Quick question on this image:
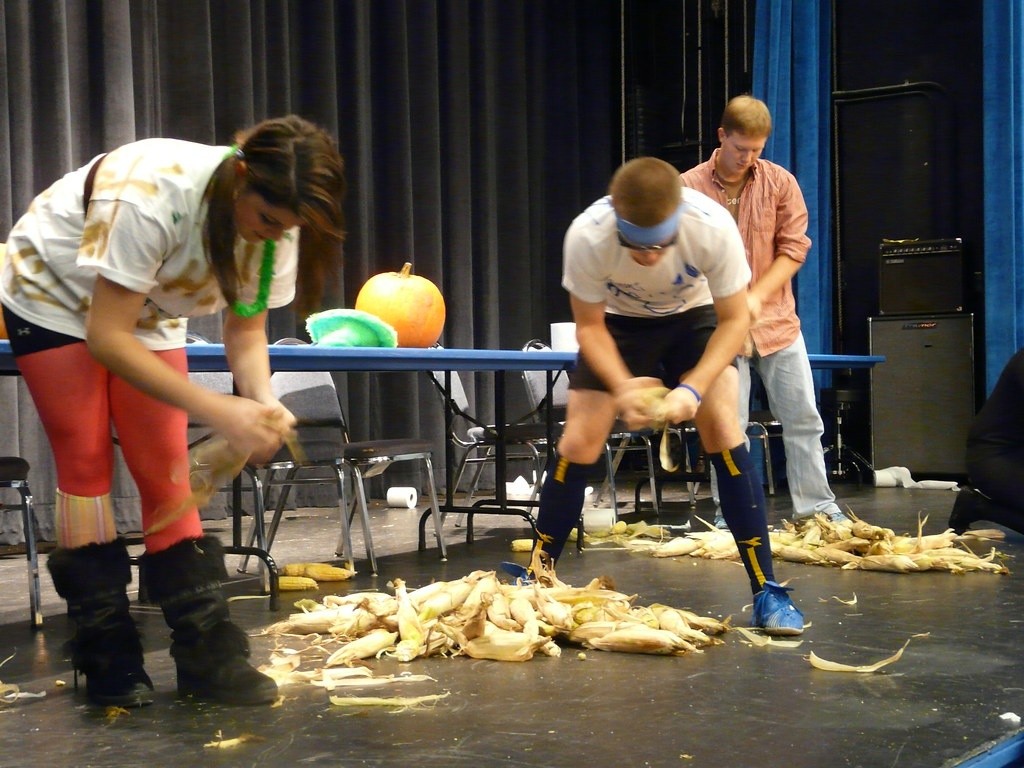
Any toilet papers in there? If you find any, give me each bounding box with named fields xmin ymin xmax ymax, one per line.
xmin=873 ymin=469 xmax=898 ymax=488
xmin=386 ymin=486 xmax=419 ymax=508
xmin=550 ymin=322 xmax=580 ymax=352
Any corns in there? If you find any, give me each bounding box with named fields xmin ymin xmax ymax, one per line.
xmin=510 ymin=516 xmax=1013 ymax=572
xmin=269 ymin=561 xmax=724 ymax=664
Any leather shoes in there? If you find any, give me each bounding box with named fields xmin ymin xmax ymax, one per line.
xmin=948 ymin=487 xmax=977 ymax=535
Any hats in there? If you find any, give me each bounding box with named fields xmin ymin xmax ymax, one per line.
xmin=305 ymin=308 xmax=398 ymax=348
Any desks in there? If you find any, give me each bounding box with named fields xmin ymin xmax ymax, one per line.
xmin=0 ymin=340 xmax=895 ymax=612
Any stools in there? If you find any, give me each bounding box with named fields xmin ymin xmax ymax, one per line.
xmin=821 ymin=385 xmax=871 ymax=491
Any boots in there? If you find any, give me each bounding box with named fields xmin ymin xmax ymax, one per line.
xmin=137 ymin=535 xmax=279 ymax=706
xmin=47 ymin=535 xmax=152 ymax=705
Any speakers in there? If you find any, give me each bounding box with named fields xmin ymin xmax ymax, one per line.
xmin=879 ymin=237 xmax=966 ymax=316
xmin=869 ymin=314 xmax=976 ymax=476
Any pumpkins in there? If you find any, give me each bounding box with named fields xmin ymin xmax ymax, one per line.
xmin=356 ymin=262 xmax=446 ymax=348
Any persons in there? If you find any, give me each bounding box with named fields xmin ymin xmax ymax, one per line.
xmin=0 ymin=115 xmax=348 ymax=709
xmin=948 ymin=347 xmax=1024 ymax=535
xmin=500 ymin=158 xmax=805 ymax=636
xmin=678 ymin=96 xmax=850 ymax=525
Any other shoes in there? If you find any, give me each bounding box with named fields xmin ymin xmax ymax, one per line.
xmin=828 ymin=511 xmax=848 ymax=523
xmin=498 ymin=561 xmax=536 ymax=585
xmin=714 ymin=516 xmax=728 ymax=528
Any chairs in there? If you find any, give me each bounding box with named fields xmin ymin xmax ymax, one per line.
xmin=0 ymin=312 xmax=872 ymax=630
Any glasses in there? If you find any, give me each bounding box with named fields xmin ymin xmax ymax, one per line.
xmin=617 ymin=231 xmax=674 ymax=251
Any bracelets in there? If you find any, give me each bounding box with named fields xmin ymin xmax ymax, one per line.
xmin=677 ymin=384 xmax=701 ymax=406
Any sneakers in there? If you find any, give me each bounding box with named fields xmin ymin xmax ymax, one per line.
xmin=752 ymin=581 xmax=803 ymax=634
